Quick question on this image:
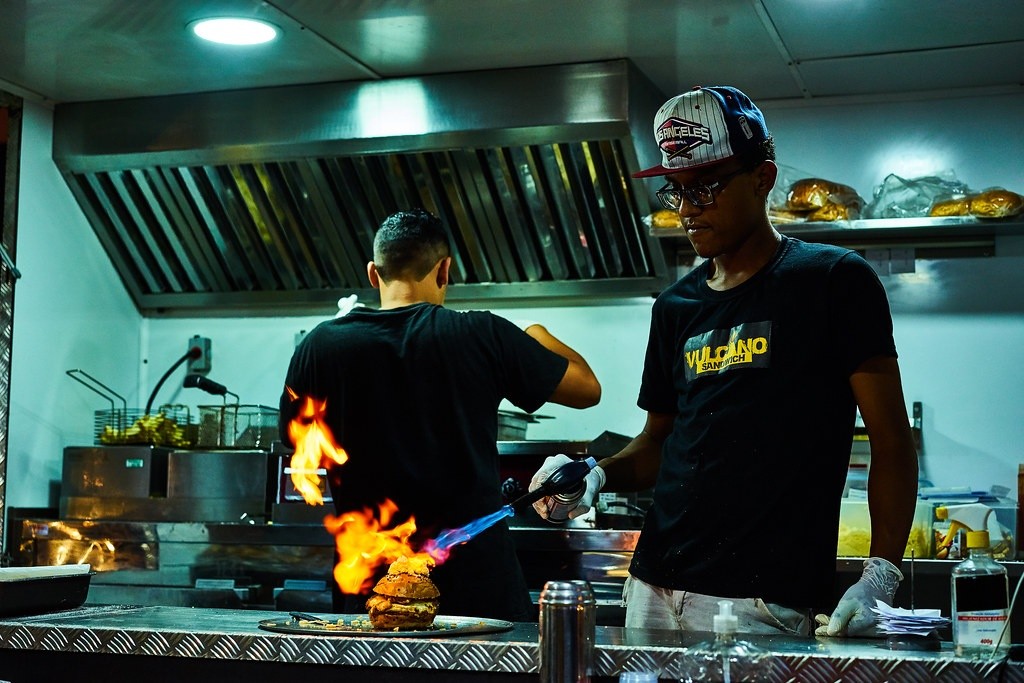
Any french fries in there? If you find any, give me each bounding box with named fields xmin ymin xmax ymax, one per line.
xmin=100 ymin=415 xmax=193 ymax=446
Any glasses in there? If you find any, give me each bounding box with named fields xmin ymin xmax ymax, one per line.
xmin=656 ymin=161 xmax=761 ymax=210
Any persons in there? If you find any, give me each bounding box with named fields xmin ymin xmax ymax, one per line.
xmin=279 ymin=209 xmax=600 ymax=622
xmin=527 ymin=87 xmax=920 ymax=638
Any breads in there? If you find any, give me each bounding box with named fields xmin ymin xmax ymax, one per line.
xmin=771 ymin=178 xmax=862 ymax=220
xmin=930 ymin=190 xmax=1024 ymax=217
xmin=653 ymin=210 xmax=681 ymax=228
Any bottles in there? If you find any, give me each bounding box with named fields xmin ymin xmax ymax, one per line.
xmin=680 ymin=600 xmax=773 ymax=683
xmin=620 ymin=672 xmax=658 ymax=683
xmin=848 ymin=464 xmax=868 ymax=491
xmin=952 ymin=532 xmax=1011 ymax=659
xmin=538 ymin=581 xmax=596 ymax=683
xmin=932 ymin=508 xmax=967 ymax=560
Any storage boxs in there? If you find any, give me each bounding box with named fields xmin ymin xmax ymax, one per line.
xmin=0 ymin=571 xmax=97 ymax=619
xmin=945 ymin=504 xmax=1021 ymax=559
xmin=834 ymin=501 xmax=936 ymax=559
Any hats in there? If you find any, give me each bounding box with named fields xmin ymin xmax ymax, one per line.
xmin=630 ymin=85 xmax=770 ymax=178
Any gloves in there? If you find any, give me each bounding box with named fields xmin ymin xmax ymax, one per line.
xmin=528 ymin=453 xmax=607 ymax=521
xmin=813 ymin=558 xmax=904 ymax=637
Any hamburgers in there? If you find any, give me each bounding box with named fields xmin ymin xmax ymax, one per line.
xmin=364 ymin=574 xmax=440 ymax=629
